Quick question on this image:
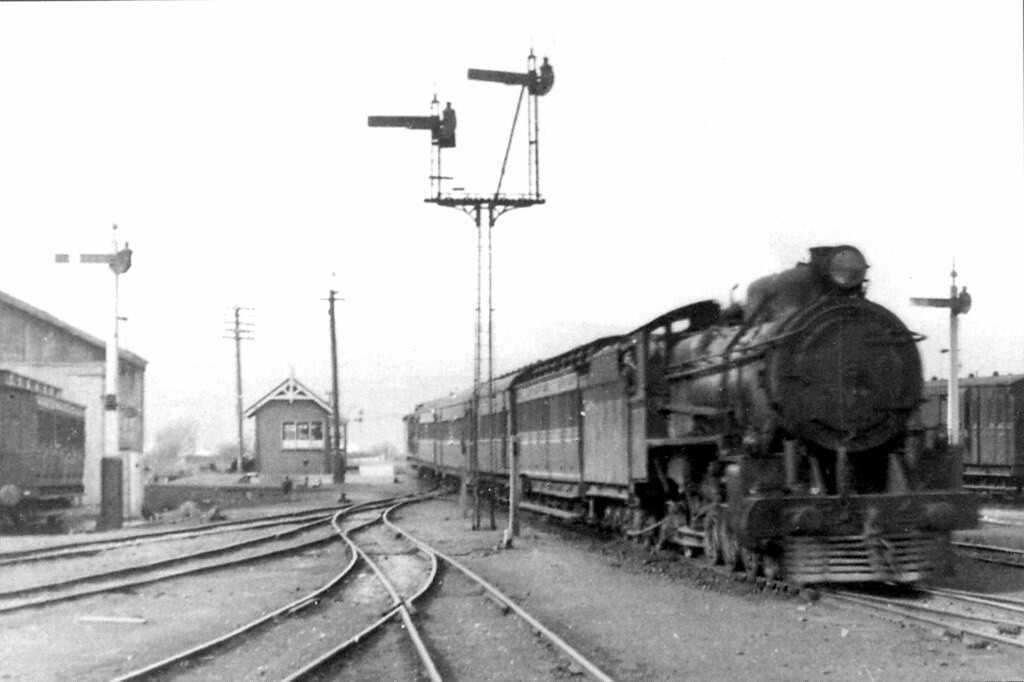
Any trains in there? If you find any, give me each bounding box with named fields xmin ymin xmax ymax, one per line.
xmin=922 ymin=372 xmax=1024 ymax=510
xmin=405 ymin=245 xmax=983 ymax=593
